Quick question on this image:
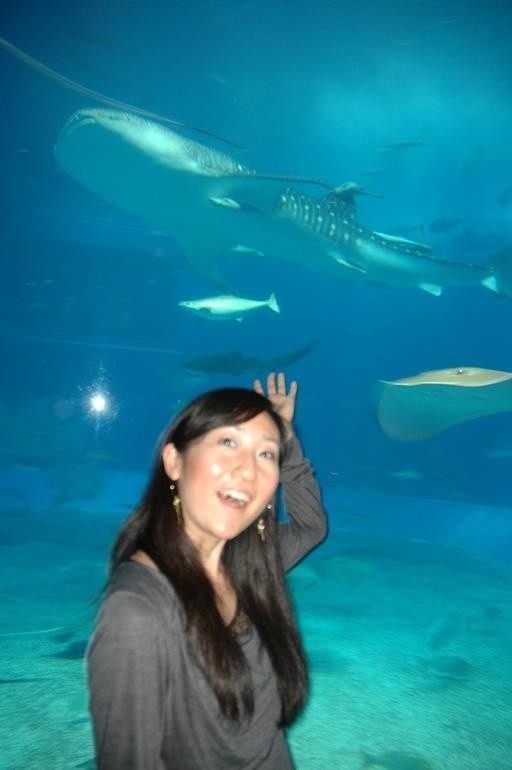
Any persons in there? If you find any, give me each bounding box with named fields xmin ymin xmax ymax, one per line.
xmin=84 ymin=373 xmax=331 ymax=770
